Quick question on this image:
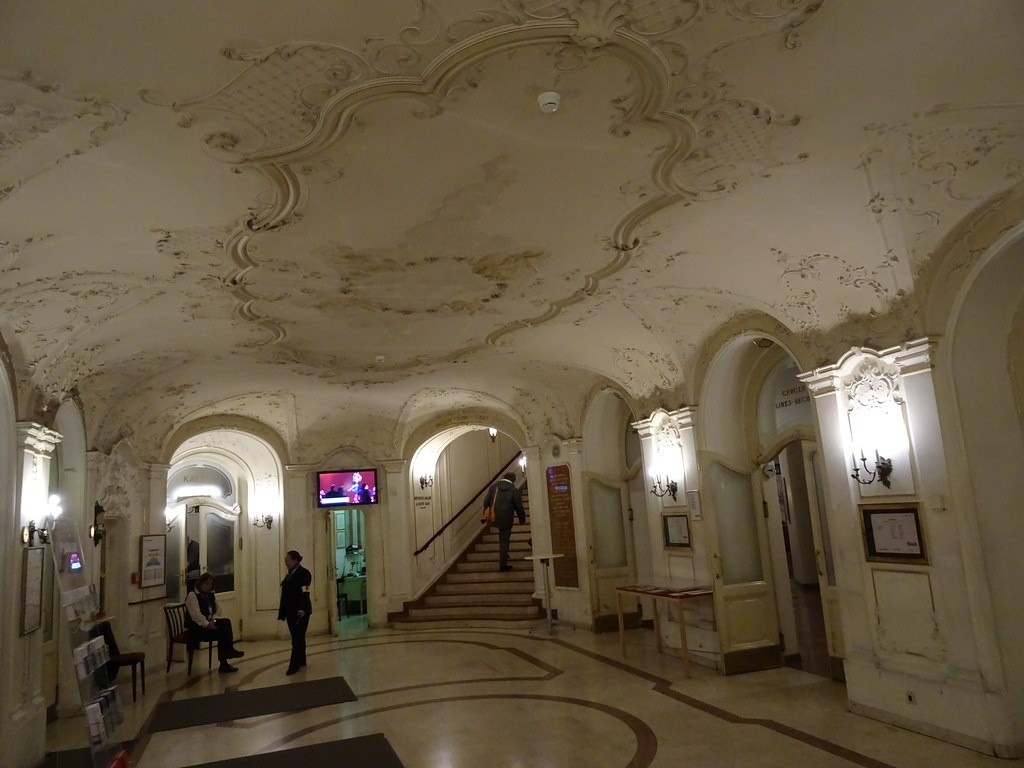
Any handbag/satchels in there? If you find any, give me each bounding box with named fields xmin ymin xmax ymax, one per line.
xmin=480 ymin=507 xmax=496 ymax=527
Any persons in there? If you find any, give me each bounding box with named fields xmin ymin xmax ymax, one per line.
xmin=184 ymin=572 xmax=245 ymax=672
xmin=278 ymin=550 xmax=312 ymax=675
xmin=325 ymin=484 xmax=337 ymax=496
xmin=334 ymin=487 xmax=346 ymax=497
xmin=187 ymin=533 xmax=199 ymax=571
xmin=357 ymin=483 xmax=371 ymax=502
xmin=483 ymin=471 xmax=526 ymax=571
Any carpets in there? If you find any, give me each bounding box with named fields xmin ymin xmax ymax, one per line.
xmin=182 ymin=732 xmax=403 ymax=768
xmin=147 ymin=676 xmax=358 ymax=734
xmin=34 ymin=739 xmax=135 ymax=768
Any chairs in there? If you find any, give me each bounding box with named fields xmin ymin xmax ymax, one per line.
xmin=163 ymin=604 xmax=212 ymax=675
xmin=87 ymin=621 xmax=146 ymax=703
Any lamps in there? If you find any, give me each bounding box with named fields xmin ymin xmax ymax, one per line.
xmin=22 ymin=495 xmax=63 ymax=545
xmin=650 ymin=473 xmax=677 ymax=502
xmin=518 ymin=456 xmax=527 ymax=480
xmin=851 ymin=448 xmax=892 ymax=489
xmin=488 ymin=427 xmax=497 ymax=444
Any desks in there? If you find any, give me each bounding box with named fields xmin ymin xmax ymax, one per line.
xmin=524 ymin=554 xmax=576 ymax=636
xmin=615 ymin=585 xmax=714 ymax=679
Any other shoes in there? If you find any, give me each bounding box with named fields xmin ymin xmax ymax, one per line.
xmin=219 ymin=664 xmax=239 ymax=673
xmin=227 ymin=648 xmax=245 ymax=659
xmin=286 ymin=665 xmax=299 ymax=675
xmin=499 ymin=565 xmax=513 ymax=572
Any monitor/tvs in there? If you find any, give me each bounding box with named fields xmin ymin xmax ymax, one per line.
xmin=316 ymin=468 xmax=378 ymax=508
